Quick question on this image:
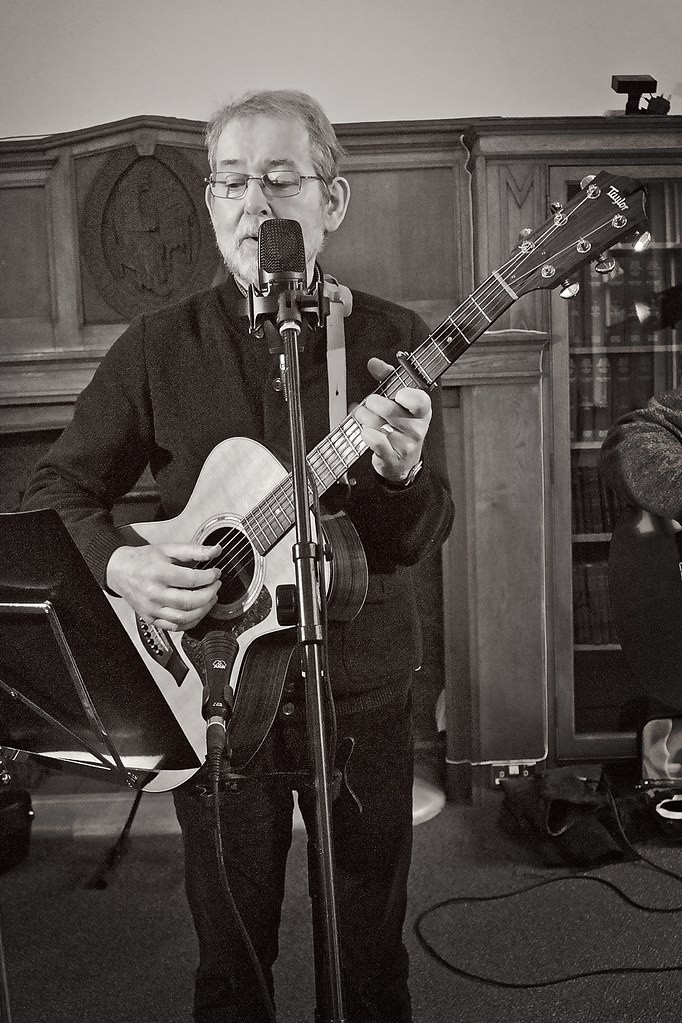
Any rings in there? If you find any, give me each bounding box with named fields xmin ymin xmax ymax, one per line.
xmin=379 ymin=424 xmax=393 ymax=437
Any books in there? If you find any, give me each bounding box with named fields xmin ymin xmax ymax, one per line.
xmin=568 ymin=258 xmax=664 ymax=648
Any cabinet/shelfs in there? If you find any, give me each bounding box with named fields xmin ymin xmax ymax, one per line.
xmin=0 ymin=112 xmax=682 ymax=812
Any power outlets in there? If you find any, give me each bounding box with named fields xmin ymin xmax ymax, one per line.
xmin=489 ymin=762 xmax=537 ymax=787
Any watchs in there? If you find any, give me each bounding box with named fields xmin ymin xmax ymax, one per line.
xmin=398 ymin=460 xmax=423 ymax=487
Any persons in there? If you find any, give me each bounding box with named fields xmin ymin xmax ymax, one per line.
xmin=598 ymin=389 xmax=682 ymax=520
xmin=19 ymin=88 xmax=455 ymax=1023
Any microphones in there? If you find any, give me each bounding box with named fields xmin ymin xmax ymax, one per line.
xmin=200 ymin=630 xmax=239 ymax=781
xmin=258 ymin=219 xmax=307 ymax=400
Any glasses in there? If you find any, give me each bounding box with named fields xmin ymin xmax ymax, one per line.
xmin=204 ymin=170 xmax=329 ymax=200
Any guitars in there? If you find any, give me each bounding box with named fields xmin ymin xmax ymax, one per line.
xmin=34 ymin=166 xmax=654 ymax=795
xmin=605 ymin=504 xmax=682 ymax=712
